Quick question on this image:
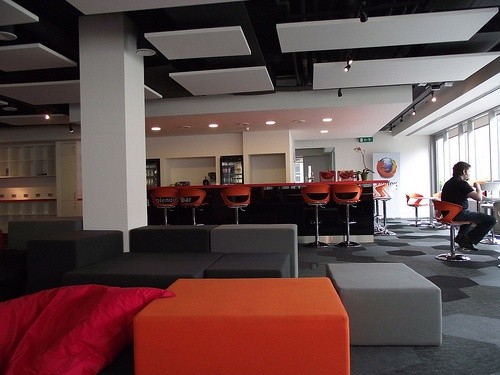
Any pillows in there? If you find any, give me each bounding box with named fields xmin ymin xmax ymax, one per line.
xmin=0 ymin=284 xmax=177 ymax=375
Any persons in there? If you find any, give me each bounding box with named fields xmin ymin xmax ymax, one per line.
xmin=441 ymin=161 xmax=496 ymax=251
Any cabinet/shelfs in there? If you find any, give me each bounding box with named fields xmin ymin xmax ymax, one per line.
xmin=0 ymin=143 xmax=57 ymax=233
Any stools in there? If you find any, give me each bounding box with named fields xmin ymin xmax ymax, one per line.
xmin=325 ymin=263 xmax=442 ymax=346
xmin=151 ymin=184 xmax=500 ymax=262
xmin=133 ymin=277 xmax=350 ymax=375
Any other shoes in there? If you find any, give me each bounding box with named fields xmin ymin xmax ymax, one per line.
xmin=458 ymin=234 xmax=478 ymax=251
xmin=454 ymin=234 xmax=466 ymax=243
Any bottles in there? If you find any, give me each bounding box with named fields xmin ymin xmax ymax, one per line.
xmin=203 ymin=177 xmax=209 ymax=186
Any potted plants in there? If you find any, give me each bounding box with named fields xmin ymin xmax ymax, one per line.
xmin=357 ymin=167 xmax=369 ymax=181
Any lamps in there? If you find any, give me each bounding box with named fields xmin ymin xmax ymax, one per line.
xmin=389 ymin=92 xmax=436 ymax=133
xmin=337 ymin=2 xmax=368 ymax=97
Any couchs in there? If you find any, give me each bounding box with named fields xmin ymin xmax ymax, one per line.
xmin=0 ymin=217 xmax=299 ymax=302
